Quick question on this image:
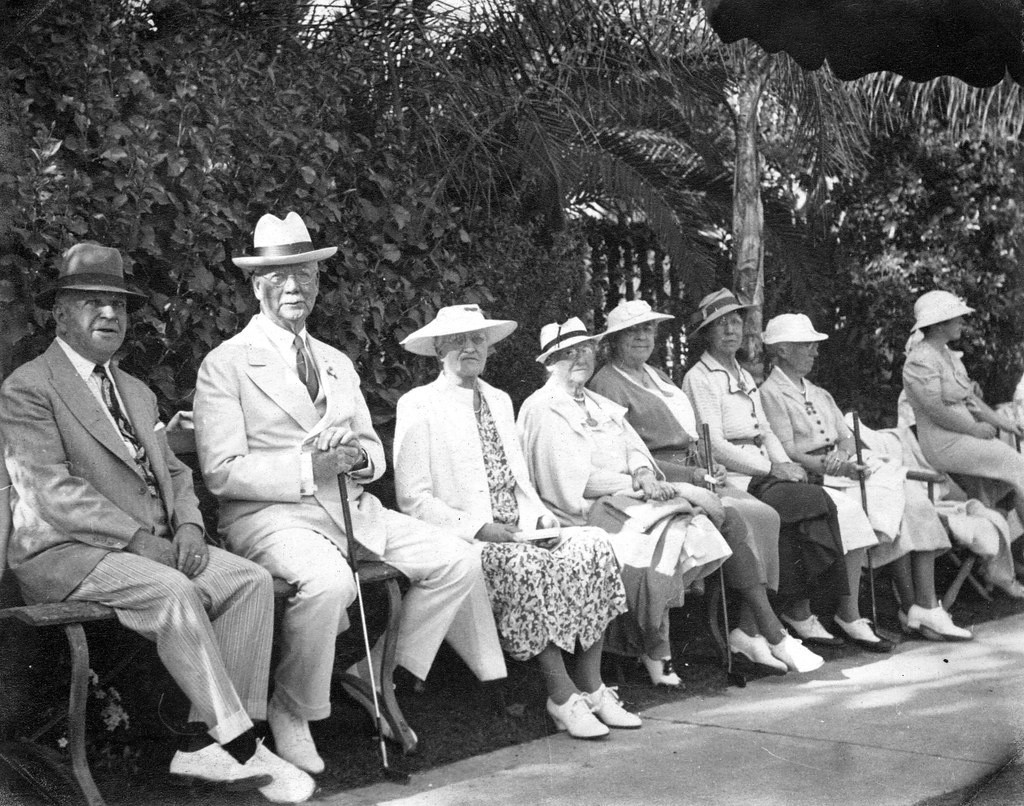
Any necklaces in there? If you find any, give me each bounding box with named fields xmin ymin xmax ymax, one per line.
xmin=475 ymin=410 xmax=481 ymax=413
xmin=799 ymin=387 xmax=805 ymax=394
xmin=573 ymin=397 xmax=584 ymax=402
xmin=641 ymin=375 xmax=649 ymax=389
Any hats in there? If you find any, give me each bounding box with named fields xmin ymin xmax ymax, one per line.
xmin=536 ymin=316 xmax=604 ymax=363
xmin=230 ymin=210 xmax=338 ymax=271
xmin=688 ymin=287 xmax=758 ymax=343
xmin=399 ymin=304 xmax=517 ymax=357
xmin=910 ymin=291 xmax=972 ymax=329
xmin=599 ymin=300 xmax=677 ymax=340
xmin=759 ymin=313 xmax=829 ymax=344
xmin=34 ymin=242 xmax=149 ymax=314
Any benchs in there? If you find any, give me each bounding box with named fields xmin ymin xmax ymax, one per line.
xmin=0 ymin=425 xmax=1024 ymax=806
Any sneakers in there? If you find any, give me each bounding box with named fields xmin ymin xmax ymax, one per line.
xmin=267 ymin=694 xmax=325 ymax=776
xmin=636 ymin=652 xmax=685 ymax=692
xmin=343 ymin=664 xmax=418 ymax=746
xmin=167 ymin=743 xmax=274 ymax=793
xmin=244 ymin=737 xmax=317 ymax=803
xmin=726 ymin=612 xmax=897 ymax=673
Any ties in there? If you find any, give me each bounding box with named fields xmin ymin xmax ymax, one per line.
xmin=93 ymin=363 xmax=161 ymax=496
xmin=293 ymin=335 xmax=320 ymax=404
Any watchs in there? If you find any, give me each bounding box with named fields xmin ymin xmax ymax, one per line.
xmin=362 ymin=449 xmax=367 ymax=463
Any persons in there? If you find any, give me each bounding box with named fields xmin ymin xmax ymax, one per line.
xmin=681 ymin=286 xmax=893 ymax=653
xmin=193 ymin=210 xmax=474 ymax=772
xmin=515 ymin=317 xmax=733 ymax=693
xmin=759 ymin=313 xmax=974 ymax=642
xmin=899 ymin=289 xmax=1024 ymax=600
xmin=393 ymin=304 xmax=643 ymax=736
xmin=1 ymin=243 xmax=316 ymax=805
xmin=587 ymin=299 xmax=822 ymax=674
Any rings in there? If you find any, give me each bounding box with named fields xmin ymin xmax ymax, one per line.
xmin=835 ymin=458 xmax=839 ymax=462
xmin=194 ymin=554 xmax=201 ymax=558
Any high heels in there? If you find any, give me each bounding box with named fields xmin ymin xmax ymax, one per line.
xmin=582 ymin=683 xmax=642 ymax=729
xmin=907 ymin=598 xmax=972 ymax=640
xmin=548 ymin=689 xmax=610 ymax=739
xmin=895 ymin=608 xmax=943 ymax=642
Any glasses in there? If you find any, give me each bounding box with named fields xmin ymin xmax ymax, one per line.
xmin=436 ymin=333 xmax=483 ymax=355
xmin=551 ymin=343 xmax=595 ymax=361
xmin=257 ymin=267 xmax=322 ymax=287
